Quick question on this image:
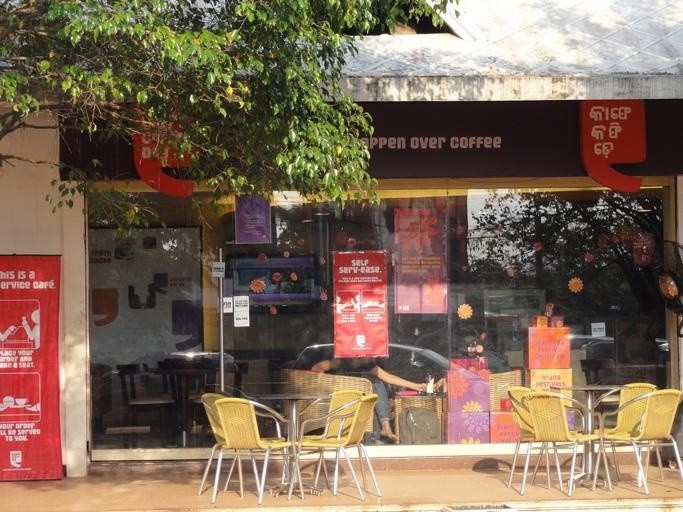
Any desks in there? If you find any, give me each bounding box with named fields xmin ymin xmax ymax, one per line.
xmin=550 ymin=383 xmax=623 ymax=495
xmin=254 ymin=393 xmax=335 ymax=495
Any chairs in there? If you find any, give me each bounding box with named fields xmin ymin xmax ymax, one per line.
xmin=592 ymin=381 xmax=659 ymax=487
xmin=303 ymin=391 xmax=369 ymax=493
xmin=506 ymin=386 xmax=585 ymax=490
xmin=600 ymin=388 xmax=683 ymax=496
xmin=296 ymin=395 xmax=382 ymax=502
xmin=518 ymin=392 xmax=593 ymax=494
xmin=213 ymin=396 xmax=296 ymax=504
xmin=199 ymin=391 xmax=286 ymax=497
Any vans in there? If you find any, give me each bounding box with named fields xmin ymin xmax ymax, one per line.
xmin=284 ymin=340 xmax=478 ymax=391
xmin=561 ymin=330 xmax=667 ymax=400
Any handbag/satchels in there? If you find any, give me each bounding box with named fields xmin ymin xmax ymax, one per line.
xmin=399 ymin=406 xmax=440 ymax=444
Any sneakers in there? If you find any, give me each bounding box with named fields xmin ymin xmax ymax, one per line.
xmin=364 ymin=439 xmax=384 ymax=445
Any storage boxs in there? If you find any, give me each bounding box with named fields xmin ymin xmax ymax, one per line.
xmin=523 ymin=326 xmax=573 ymax=370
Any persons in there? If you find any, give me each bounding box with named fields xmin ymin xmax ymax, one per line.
xmin=310 ymin=357 xmax=428 ymax=444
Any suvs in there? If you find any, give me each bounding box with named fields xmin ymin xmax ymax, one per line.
xmin=99 ymin=351 xmax=236 ymax=403
